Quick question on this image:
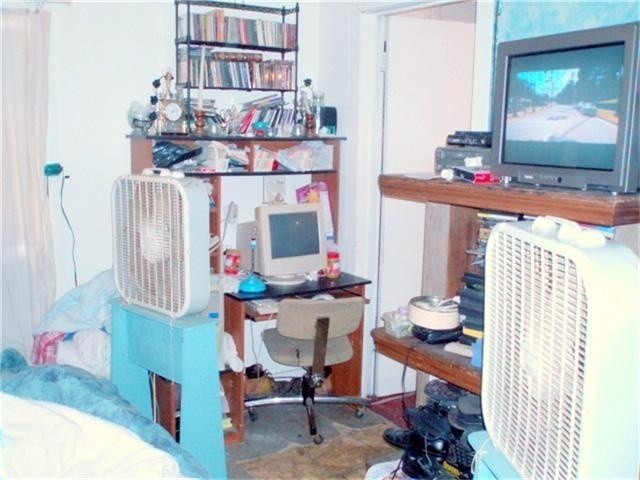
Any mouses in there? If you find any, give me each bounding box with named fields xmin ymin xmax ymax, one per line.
xmin=311 ymin=293 xmax=335 ymax=301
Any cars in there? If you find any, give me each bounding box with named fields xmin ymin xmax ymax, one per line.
xmin=575 ymin=101 xmax=584 ymax=111
xmin=580 ymin=102 xmax=598 ymax=117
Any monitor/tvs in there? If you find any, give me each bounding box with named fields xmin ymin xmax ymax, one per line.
xmin=489 ymin=20 xmax=640 ymax=196
xmin=236 ymin=201 xmax=328 ymax=285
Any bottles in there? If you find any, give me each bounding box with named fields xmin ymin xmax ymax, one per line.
xmin=223 ymin=248 xmax=241 ymax=295
xmin=326 ymin=236 xmax=342 ymax=280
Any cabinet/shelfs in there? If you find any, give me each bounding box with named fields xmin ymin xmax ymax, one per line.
xmin=172 ymin=2 xmax=301 ymax=130
xmin=132 ymin=132 xmax=346 ymax=281
xmin=151 ymin=273 xmax=372 ymax=445
xmin=374 ymin=170 xmax=640 ymax=461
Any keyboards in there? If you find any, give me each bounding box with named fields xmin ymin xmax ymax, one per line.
xmin=252 ymin=295 xmax=302 ymax=316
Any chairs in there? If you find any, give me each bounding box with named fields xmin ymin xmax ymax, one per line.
xmin=245 ymin=297 xmax=369 ymax=444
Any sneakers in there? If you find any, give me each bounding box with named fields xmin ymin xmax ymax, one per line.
xmin=282 ymin=366 xmax=332 ymax=396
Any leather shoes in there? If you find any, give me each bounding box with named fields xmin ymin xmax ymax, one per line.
xmin=384 ymin=403 xmax=457 ymax=480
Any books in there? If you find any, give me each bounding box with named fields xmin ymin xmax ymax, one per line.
xmin=455 ymin=208 xmax=524 ymax=349
xmin=179 ymin=8 xmax=298 ymax=136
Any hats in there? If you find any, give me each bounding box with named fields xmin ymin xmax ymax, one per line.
xmin=448 ymin=396 xmax=484 ymax=431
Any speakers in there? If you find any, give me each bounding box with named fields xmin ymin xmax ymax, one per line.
xmin=320 ymin=106 xmax=337 ymax=134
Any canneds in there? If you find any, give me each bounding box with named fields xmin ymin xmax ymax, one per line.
xmin=327 ymin=252 xmax=341 ymax=279
